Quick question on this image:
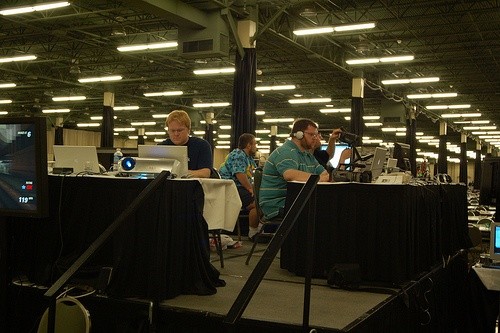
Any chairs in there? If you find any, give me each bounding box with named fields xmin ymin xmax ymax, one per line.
xmin=468 ymin=188 xmax=494 ymax=259
xmin=245 ymin=167 xmax=283 ymax=265
xmin=211 ymin=168 xmax=248 ymax=241
xmin=37 ymin=296 xmax=92 ymax=333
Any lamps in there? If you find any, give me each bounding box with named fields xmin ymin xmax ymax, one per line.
xmin=0 ymin=0 xmax=500 ymax=163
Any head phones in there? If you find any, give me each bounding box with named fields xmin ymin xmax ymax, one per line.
xmin=290 ymin=131 xmax=303 ymax=140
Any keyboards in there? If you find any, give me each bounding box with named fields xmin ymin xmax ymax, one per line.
xmin=482 ymin=265 xmax=500 ymax=268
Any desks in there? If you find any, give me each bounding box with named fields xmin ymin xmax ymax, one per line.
xmin=471 ymin=260 xmax=500 ymax=333
xmin=468 ymin=216 xmax=493 ymax=220
xmin=473 ymin=223 xmax=491 ymax=241
xmin=28 ymin=173 xmax=226 ymax=302
xmin=468 ymin=206 xmax=496 ymax=210
xmin=280 ymin=181 xmax=467 ymax=286
xmin=178 ymin=177 xmax=242 ymax=268
xmin=479 ymin=210 xmax=496 ymax=214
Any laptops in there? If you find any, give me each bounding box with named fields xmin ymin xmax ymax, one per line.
xmin=320 ymin=142 xmax=348 ymax=168
xmin=53 ymin=144 xmax=105 ymax=175
xmin=138 ymin=144 xmax=189 ymax=178
xmin=358 ymin=147 xmax=387 ymax=172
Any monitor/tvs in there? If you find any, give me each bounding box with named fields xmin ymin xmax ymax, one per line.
xmin=487 ymin=222 xmax=500 ymax=263
xmin=0 ymin=118 xmax=49 ymax=218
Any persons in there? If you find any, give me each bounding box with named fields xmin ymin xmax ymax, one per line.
xmin=312 ymin=129 xmax=343 ymax=168
xmin=219 ymin=134 xmax=258 ymax=240
xmin=158 ymin=110 xmax=213 ymax=178
xmin=259 ymin=119 xmax=329 ymax=220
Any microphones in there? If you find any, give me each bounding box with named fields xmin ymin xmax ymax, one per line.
xmin=338 ymin=133 xmax=354 ymax=144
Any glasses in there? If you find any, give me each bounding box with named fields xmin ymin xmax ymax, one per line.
xmin=303 ymin=131 xmax=318 ymax=137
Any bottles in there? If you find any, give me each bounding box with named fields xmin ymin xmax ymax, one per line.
xmin=113 ymin=148 xmax=123 ymax=175
